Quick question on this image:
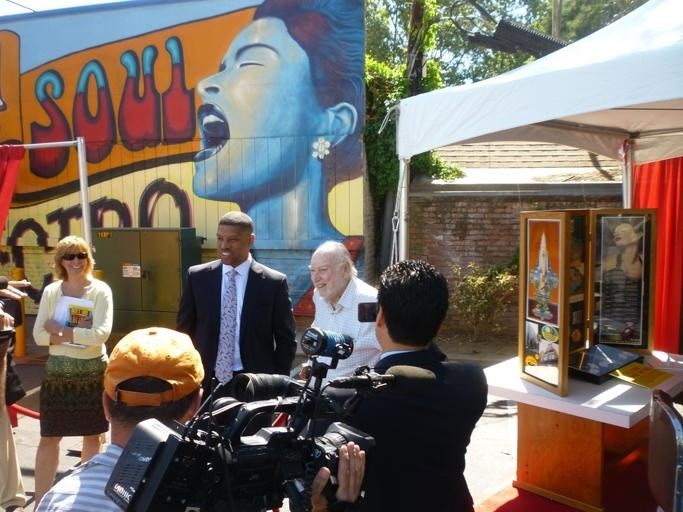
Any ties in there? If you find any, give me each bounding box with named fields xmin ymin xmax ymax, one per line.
xmin=215 ymin=270 xmax=237 ymax=386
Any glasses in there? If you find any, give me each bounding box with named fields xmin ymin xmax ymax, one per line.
xmin=62 ymin=252 xmax=88 ymax=260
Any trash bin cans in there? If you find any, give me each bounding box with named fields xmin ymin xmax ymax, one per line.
xmin=1 ymin=301 xmax=23 ymax=328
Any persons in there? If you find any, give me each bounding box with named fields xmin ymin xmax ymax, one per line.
xmin=188 ymin=0 xmax=365 ymax=251
xmin=35 ymin=326 xmax=367 ymax=512
xmin=33 ymin=235 xmax=114 ymax=512
xmin=176 ymin=209 xmax=298 ymax=398
xmin=290 ymin=240 xmax=384 ymax=388
xmin=326 ymin=260 xmax=489 ymax=512
xmin=601 ymin=221 xmax=644 ymax=328
xmin=0 ymin=271 xmax=31 ymax=512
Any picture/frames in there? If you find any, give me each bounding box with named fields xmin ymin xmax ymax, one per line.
xmin=515 ymin=206 xmax=661 ymax=398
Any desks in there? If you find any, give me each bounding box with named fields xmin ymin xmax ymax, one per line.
xmin=481 ymin=347 xmax=683 ymax=511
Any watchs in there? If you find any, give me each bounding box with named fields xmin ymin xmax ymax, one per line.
xmin=57 ymin=324 xmax=68 ymax=339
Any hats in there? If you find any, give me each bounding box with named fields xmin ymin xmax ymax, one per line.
xmin=104 ymin=325 xmax=206 ymax=408
